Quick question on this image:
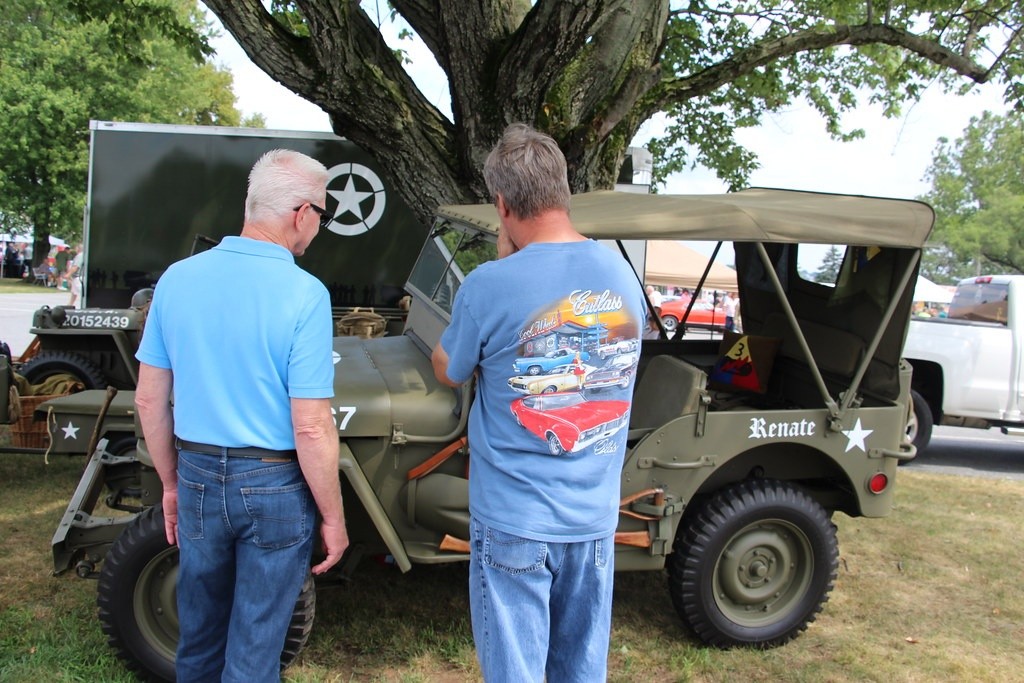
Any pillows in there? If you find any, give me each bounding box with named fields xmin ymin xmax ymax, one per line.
xmin=711 ymin=329 xmax=785 ymax=395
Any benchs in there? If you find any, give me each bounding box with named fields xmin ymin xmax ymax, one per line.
xmin=679 ymin=354 xmax=722 ymax=374
xmin=743 ymin=313 xmax=864 ymax=407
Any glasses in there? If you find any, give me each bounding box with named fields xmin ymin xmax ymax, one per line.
xmin=294 ymin=203 xmax=335 ymax=228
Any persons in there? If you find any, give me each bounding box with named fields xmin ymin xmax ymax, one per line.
xmin=134 ymin=148 xmax=349 ymax=683
xmin=913 ymin=301 xmax=950 ymax=318
xmin=645 ymin=286 xmax=742 ymax=334
xmin=430 ymin=123 xmax=648 ymax=683
xmin=0 ymin=241 xmax=83 ymax=306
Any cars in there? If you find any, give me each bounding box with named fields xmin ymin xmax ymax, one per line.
xmin=41 ymin=176 xmax=942 ymax=683
xmin=646 ymin=297 xmax=728 ymax=332
xmin=12 ymin=119 xmax=448 ymax=423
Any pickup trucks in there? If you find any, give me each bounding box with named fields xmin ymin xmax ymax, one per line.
xmin=897 ymin=274 xmax=1024 ymax=467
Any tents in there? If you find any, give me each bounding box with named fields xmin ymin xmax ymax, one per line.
xmin=913 ymin=274 xmax=955 ymax=302
xmin=646 ymin=239 xmax=739 ymax=292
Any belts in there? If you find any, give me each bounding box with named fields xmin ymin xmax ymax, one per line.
xmin=181 ymin=440 xmax=297 ymax=459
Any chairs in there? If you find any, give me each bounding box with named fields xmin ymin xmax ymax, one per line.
xmin=32 ymin=267 xmax=47 ymax=286
xmin=622 ymin=353 xmax=710 ymax=462
